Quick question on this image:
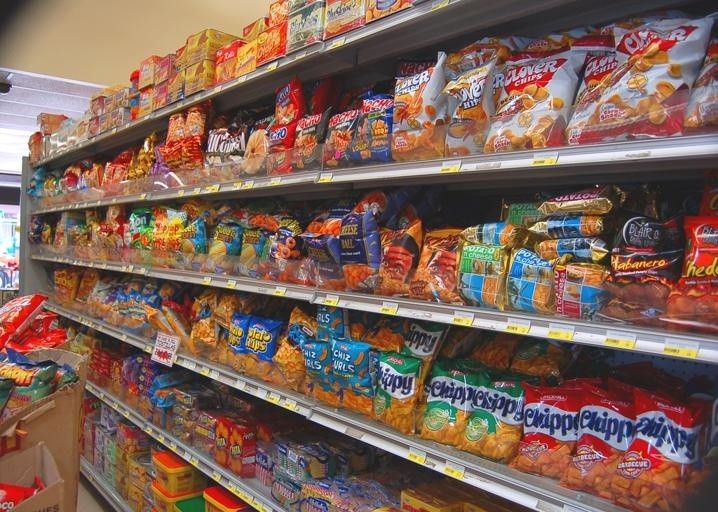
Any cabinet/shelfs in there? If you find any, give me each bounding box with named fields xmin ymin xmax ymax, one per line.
xmin=17 ymin=1 xmax=718 ymax=512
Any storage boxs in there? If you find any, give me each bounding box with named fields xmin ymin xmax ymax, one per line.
xmin=0 ymin=351 xmax=88 ymax=512
xmin=0 ymin=441 xmax=65 ymax=512
xmin=78 ymin=394 xmax=257 ymax=512
xmin=184 ymin=1 xmax=414 ymax=99
